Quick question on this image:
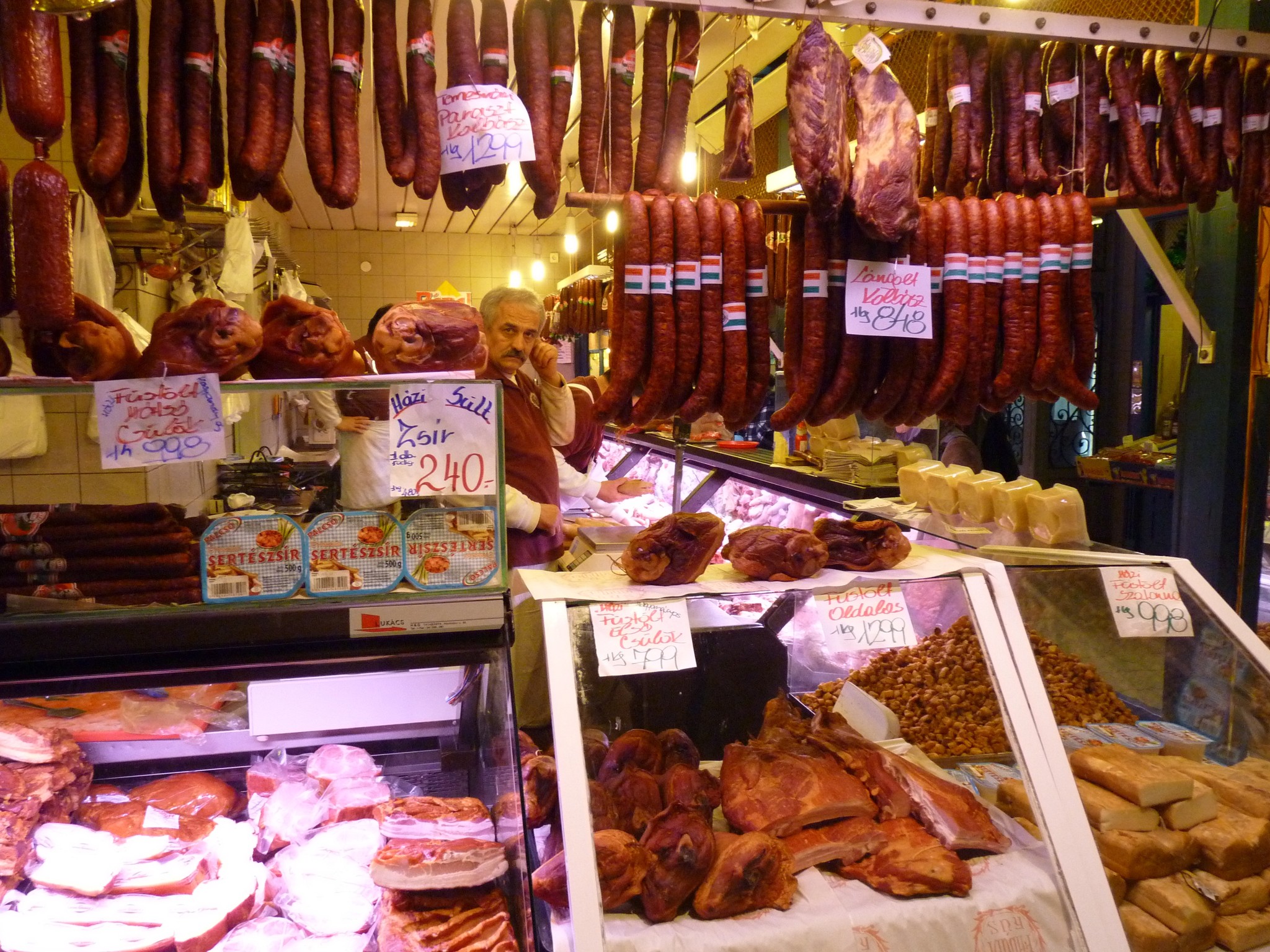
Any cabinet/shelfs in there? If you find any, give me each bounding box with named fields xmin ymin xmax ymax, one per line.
xmin=216 ymin=456 xmax=302 ymax=505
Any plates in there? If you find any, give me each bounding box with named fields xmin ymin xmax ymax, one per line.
xmin=274 ymin=507 xmax=309 ymax=515
xmin=716 ymin=441 xmax=760 ymax=449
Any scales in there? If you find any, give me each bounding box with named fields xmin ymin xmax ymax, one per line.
xmin=559 ymin=417 xmax=691 ymax=578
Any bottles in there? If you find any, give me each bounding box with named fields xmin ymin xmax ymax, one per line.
xmin=1173 ymin=409 xmax=1179 ymax=437
xmin=1161 ymin=401 xmax=1174 ymax=437
xmin=795 ymin=422 xmax=809 ymax=452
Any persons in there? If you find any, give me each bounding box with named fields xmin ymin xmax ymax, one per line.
xmin=556 ymin=365 xmax=645 ymax=529
xmin=741 ymin=395 xmax=775 ymax=451
xmin=766 ymin=373 xmax=776 ymax=401
xmin=473 ymin=288 xmax=578 ymax=569
xmin=354 ymin=303 xmax=394 ymax=377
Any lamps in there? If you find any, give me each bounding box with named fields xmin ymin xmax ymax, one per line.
xmin=394 ymin=212 xmax=419 ymax=228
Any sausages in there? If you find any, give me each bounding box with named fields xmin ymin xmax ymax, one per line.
xmin=0 ymin=3 xmax=1268 ymax=622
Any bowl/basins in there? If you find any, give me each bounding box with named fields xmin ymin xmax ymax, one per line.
xmin=804 ymin=417 xmax=1093 ymax=549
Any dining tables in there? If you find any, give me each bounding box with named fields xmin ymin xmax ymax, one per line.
xmin=207 ymin=489 xmax=317 ymax=525
xmin=276 ymin=435 xmax=340 ymax=512
